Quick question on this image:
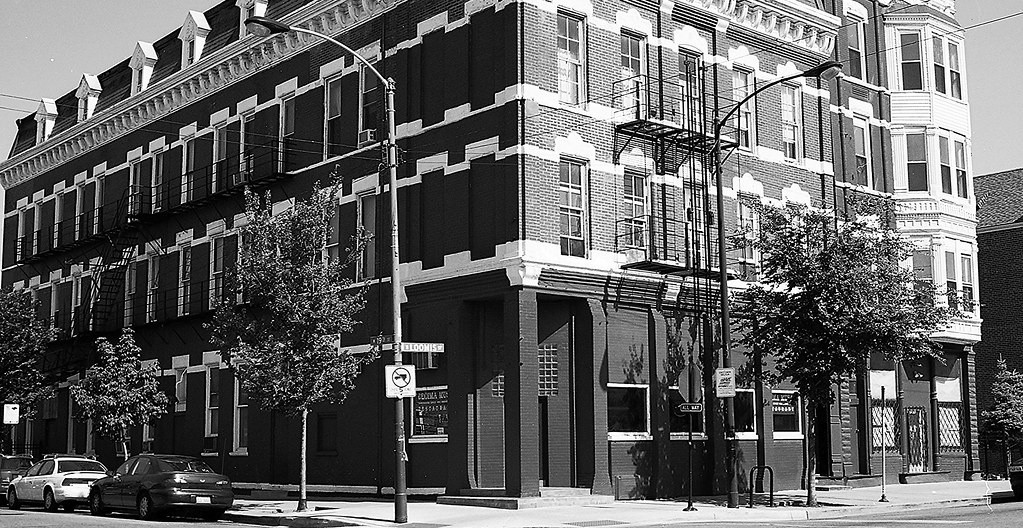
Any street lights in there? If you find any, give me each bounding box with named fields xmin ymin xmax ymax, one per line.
xmin=245 ymin=15 xmax=408 ymax=524
xmin=711 ymin=60 xmax=846 ymax=507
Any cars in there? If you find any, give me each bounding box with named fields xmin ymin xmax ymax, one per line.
xmin=4 ymin=452 xmax=109 ymax=512
xmin=88 ymin=454 xmax=235 ymax=522
xmin=0 ymin=452 xmax=35 ymax=495
